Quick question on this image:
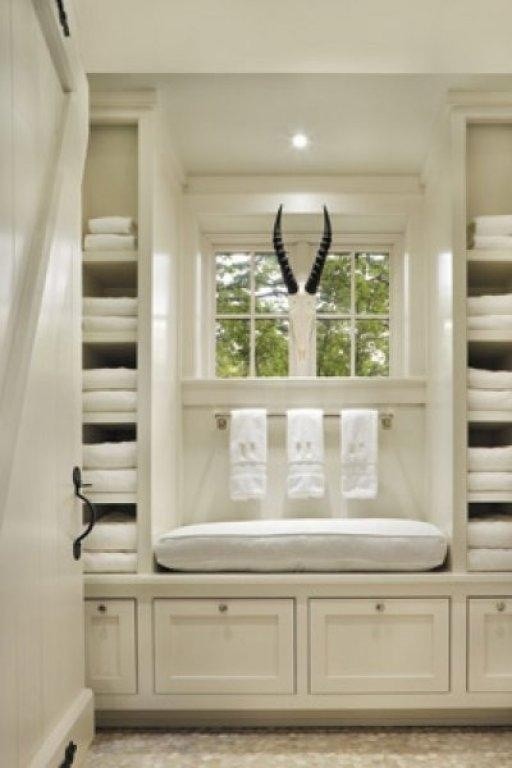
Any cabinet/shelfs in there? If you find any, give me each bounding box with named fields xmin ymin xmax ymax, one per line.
xmin=84 ymin=575 xmax=512 ymax=729
xmin=73 ymin=85 xmax=149 ymax=577
xmin=452 ymin=70 xmax=512 ymax=576
